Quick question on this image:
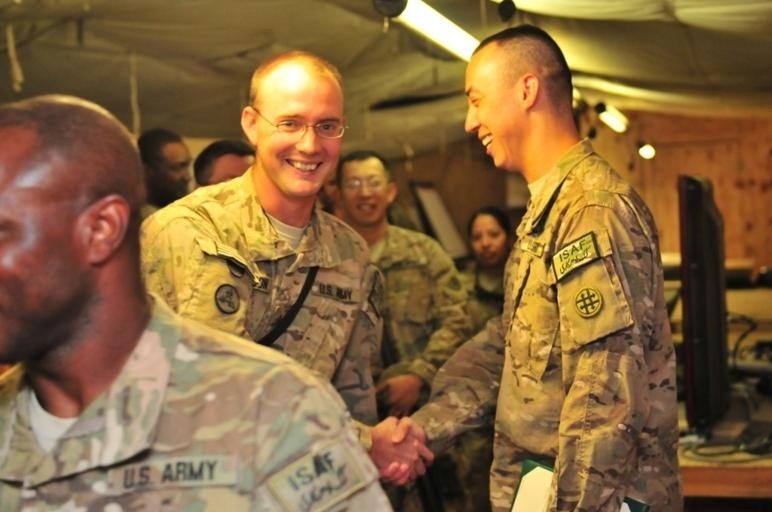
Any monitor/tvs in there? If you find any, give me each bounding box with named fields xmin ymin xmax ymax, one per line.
xmin=677 ymin=174 xmax=728 ymax=430
xmin=407 ymin=179 xmax=475 ymax=271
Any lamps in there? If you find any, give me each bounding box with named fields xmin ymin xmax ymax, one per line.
xmin=372 ymin=1 xmax=482 ymax=63
xmin=592 ymin=99 xmax=633 ymax=137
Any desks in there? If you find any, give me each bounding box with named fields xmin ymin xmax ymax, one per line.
xmin=665 ymin=284 xmax=772 ymax=498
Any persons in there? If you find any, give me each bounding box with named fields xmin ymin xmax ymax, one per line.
xmin=0 ymin=92 xmax=395 ymax=512
xmin=382 ymin=25 xmax=683 ymax=512
xmin=139 ymin=51 xmax=434 ymax=489
xmin=139 ymin=129 xmax=255 ymax=221
xmin=316 ymin=152 xmax=511 ymax=512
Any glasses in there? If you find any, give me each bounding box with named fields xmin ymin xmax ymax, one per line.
xmin=252 ymin=107 xmax=349 ymax=139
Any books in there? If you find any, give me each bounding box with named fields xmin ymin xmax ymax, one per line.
xmin=511 ymin=456 xmax=650 ymax=512
